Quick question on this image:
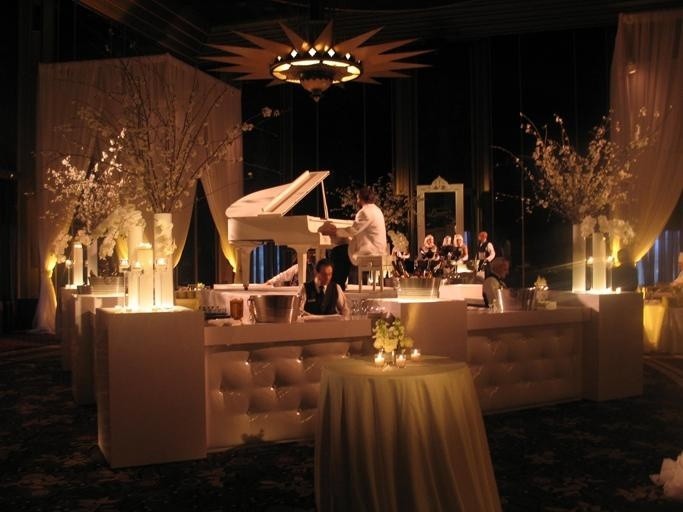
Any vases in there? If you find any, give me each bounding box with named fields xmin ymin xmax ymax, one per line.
xmin=384 ymin=339 xmax=398 ymax=353
xmin=573 ymin=222 xmax=608 ymax=295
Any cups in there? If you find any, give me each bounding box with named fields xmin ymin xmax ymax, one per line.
xmin=231 ymin=298 xmax=243 ymax=319
xmin=243 ymin=281 xmax=249 ymax=290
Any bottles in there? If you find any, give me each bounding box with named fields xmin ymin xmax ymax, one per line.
xmin=341 ymin=297 xmax=366 ymax=319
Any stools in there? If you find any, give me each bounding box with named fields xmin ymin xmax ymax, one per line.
xmin=359 ymin=254 xmax=396 ymax=291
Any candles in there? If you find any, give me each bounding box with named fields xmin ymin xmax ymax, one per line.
xmin=374 ymin=350 xmax=422 ymax=367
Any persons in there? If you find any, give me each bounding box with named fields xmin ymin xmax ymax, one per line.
xmin=482 ymin=257 xmax=510 ymax=308
xmin=474 ymin=231 xmax=496 ymax=271
xmin=296 ymin=258 xmax=350 ymax=317
xmin=611 ymin=249 xmax=638 ymax=291
xmin=669 ymin=252 xmax=683 ymax=293
xmin=317 ymin=188 xmax=386 ymax=291
xmin=394 ymin=246 xmax=413 ymax=273
xmin=420 ymin=233 xmax=469 ymax=264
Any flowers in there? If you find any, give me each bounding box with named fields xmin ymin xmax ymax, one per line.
xmin=489 ymin=100 xmax=677 ymax=238
xmin=373 ymin=318 xmax=405 ymax=347
xmin=21 ymin=32 xmax=279 ymax=308
xmin=328 ymin=174 xmax=423 ymax=231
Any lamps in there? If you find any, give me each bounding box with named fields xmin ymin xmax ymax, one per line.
xmin=269 ymin=45 xmax=364 ymax=104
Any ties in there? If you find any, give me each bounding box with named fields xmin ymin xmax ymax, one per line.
xmin=319 ymin=285 xmax=325 ymax=295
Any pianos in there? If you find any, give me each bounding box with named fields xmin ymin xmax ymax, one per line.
xmin=225 ymin=170 xmax=354 ymax=291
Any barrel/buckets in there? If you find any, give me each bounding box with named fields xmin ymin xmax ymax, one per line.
xmin=498 ymin=286 xmax=536 ymax=312
xmin=395 ymin=275 xmax=442 ymax=298
xmin=86 ymin=276 xmax=126 ymax=295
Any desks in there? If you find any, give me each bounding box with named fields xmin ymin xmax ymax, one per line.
xmin=317 ymin=350 xmax=502 ymax=512
xmin=642 ymin=298 xmax=681 ymax=357
